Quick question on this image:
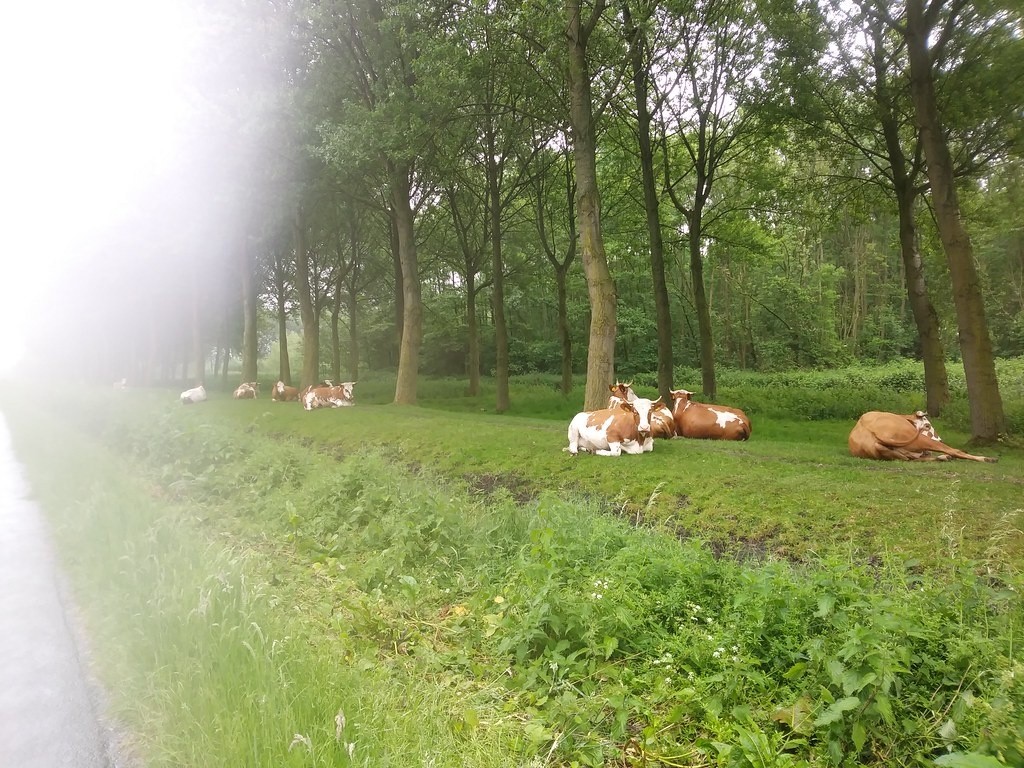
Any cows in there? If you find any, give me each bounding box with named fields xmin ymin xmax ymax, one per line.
xmin=562 ymin=396 xmax=663 ymax=456
xmin=669 ymin=387 xmax=752 ymax=442
xmin=848 ymin=410 xmax=999 ymax=463
xmin=297 ymin=380 xmax=357 ymax=411
xmin=609 ymin=379 xmax=676 ymax=457
xmin=233 ymin=382 xmax=261 ymax=399
xmin=181 ymin=386 xmax=206 ymax=405
xmin=272 ymin=380 xmax=300 ymax=402
